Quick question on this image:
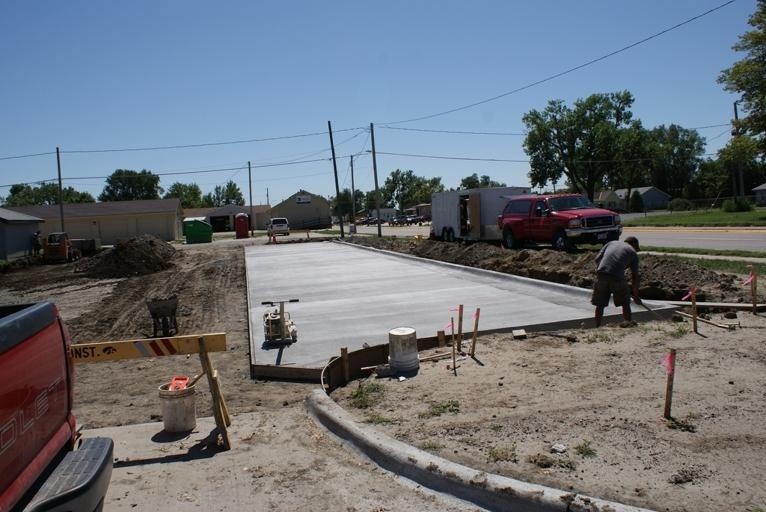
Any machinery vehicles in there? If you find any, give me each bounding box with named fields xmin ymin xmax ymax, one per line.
xmin=42 ymin=231 xmax=80 ymax=265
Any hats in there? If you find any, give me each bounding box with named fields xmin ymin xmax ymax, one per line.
xmin=624 ymin=236 xmax=642 ymax=252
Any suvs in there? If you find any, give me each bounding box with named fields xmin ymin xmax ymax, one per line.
xmin=266 ymin=217 xmax=289 ymax=236
xmin=497 ymin=192 xmax=622 ymax=254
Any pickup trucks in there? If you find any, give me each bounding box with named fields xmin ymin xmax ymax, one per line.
xmin=0 ymin=299 xmax=115 ymax=511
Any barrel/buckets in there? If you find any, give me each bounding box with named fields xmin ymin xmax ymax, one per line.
xmin=157 ymin=381 xmax=197 ymax=434
xmin=388 ymin=326 xmax=420 ymax=372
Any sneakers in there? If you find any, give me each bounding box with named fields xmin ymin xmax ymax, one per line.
xmin=594 ymin=319 xmax=639 ymax=328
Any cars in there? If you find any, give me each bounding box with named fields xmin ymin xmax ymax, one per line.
xmin=354 ymin=214 xmax=430 ymax=226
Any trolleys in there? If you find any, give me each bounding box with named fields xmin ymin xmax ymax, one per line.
xmin=146 ymin=295 xmax=179 ymax=337
xmin=260 ymin=298 xmax=299 ymax=344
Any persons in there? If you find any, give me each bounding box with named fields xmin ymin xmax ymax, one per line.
xmin=31 ymin=229 xmax=41 ymax=258
xmin=591 ymin=235 xmax=643 ymax=328
